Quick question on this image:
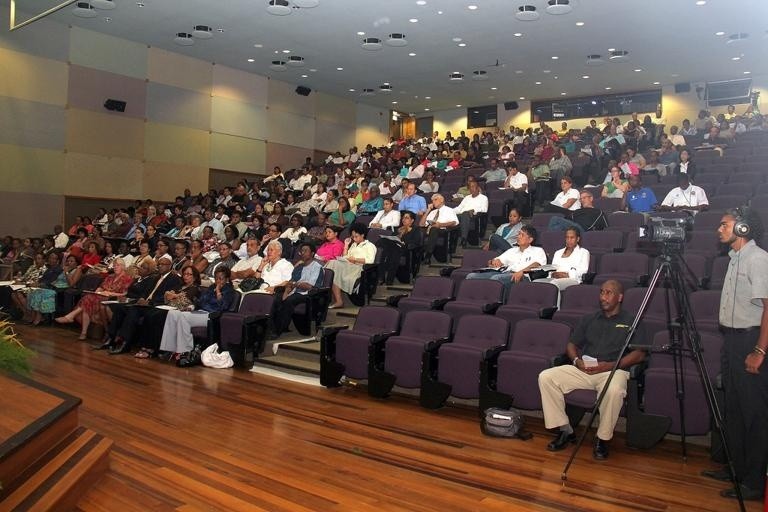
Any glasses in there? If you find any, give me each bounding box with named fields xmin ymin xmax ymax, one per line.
xmin=158 ymin=263 xmax=170 ymax=265
xmin=579 ymin=195 xmax=592 ymax=202
xmin=183 ymin=273 xmax=192 ymax=276
xmin=175 ymin=247 xmax=184 ymax=250
xmin=268 ymin=229 xmax=277 ymax=232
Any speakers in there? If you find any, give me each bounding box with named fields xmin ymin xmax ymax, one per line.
xmin=104 ymin=99 xmax=126 ymax=112
xmin=296 ymin=87 xmax=311 ymax=96
xmin=675 ymin=83 xmax=690 ymax=92
xmin=504 ymin=101 xmax=518 ymax=110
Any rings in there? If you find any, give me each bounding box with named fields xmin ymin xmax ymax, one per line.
xmin=745 ymin=367 xmax=749 ymax=371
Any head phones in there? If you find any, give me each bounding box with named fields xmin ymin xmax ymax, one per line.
xmin=733 ymin=207 xmax=752 ymax=237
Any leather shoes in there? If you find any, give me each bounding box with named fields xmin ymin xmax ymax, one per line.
xmin=91 ymin=332 xmax=115 ymax=350
xmin=704 ymin=466 xmax=731 ymax=483
xmin=720 ymin=484 xmax=765 ymax=502
xmin=55 ymin=317 xmax=74 ymax=323
xmin=107 ymin=339 xmax=131 ymax=354
xmin=328 ymin=302 xmax=344 ymax=309
xmin=79 ymin=331 xmax=87 ymax=339
xmin=547 ymin=427 xmax=577 ymax=451
xmin=592 ymin=433 xmax=609 ymax=460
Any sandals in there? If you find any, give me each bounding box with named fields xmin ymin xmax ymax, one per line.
xmin=134 ymin=348 xmax=146 ymax=358
xmin=139 ymin=350 xmax=155 ymax=359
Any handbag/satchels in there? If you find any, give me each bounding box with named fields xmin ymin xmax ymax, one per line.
xmin=201 ymin=343 xmax=234 ymax=369
xmin=238 ymin=276 xmax=264 ymax=292
xmin=480 ymin=406 xmax=521 ymax=437
xmin=177 ymin=344 xmax=201 ymax=367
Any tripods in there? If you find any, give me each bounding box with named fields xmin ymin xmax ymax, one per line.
xmin=561 ymin=255 xmax=746 ymax=511
xmin=743 ymin=99 xmax=760 ymax=116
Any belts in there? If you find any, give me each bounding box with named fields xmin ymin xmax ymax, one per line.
xmin=719 ymin=325 xmax=761 ymax=334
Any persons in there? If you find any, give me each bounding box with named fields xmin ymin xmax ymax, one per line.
xmin=700 ymin=204 xmax=768 ymax=504
xmin=1 ymin=101 xmax=768 ymax=366
xmin=537 ymin=279 xmax=647 ymax=461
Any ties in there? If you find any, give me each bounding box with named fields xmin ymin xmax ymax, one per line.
xmin=425 ymin=209 xmax=439 ymax=235
